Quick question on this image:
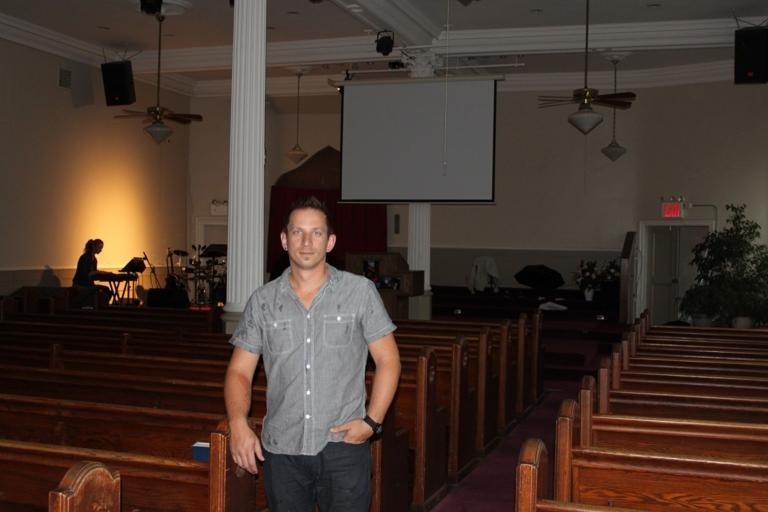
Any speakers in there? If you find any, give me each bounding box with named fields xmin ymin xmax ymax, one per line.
xmin=733 ymin=26 xmax=767 ymax=85
xmin=101 ymin=60 xmax=137 ymax=106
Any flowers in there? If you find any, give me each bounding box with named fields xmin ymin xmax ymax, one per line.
xmin=576 ymin=257 xmax=602 ymax=286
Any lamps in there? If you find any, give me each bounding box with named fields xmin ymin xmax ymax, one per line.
xmin=568 ymin=107 xmax=605 ymax=136
xmin=286 ymin=75 xmax=309 ymax=166
xmin=601 ymin=53 xmax=627 ymax=162
xmin=145 ymin=120 xmax=171 ymax=144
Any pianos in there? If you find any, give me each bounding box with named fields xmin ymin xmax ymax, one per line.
xmin=99 ymin=272 xmax=139 ymax=283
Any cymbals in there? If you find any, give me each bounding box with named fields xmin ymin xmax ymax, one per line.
xmin=171 ymin=249 xmax=189 ymax=257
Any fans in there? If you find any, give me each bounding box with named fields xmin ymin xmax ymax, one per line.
xmin=113 ymin=12 xmax=204 ymax=127
xmin=536 ymin=2 xmax=636 ymax=109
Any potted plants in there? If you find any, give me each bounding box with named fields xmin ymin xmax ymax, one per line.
xmin=680 ymin=202 xmax=768 ymax=329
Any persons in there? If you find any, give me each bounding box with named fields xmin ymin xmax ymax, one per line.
xmin=224 ymin=196 xmax=401 ymax=511
xmin=72 ymin=239 xmax=109 ymax=293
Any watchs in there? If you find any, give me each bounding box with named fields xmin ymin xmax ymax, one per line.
xmin=363 ymin=415 xmax=384 ymax=435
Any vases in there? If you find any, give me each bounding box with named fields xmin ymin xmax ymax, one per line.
xmin=585 ymin=286 xmax=595 ymax=302
